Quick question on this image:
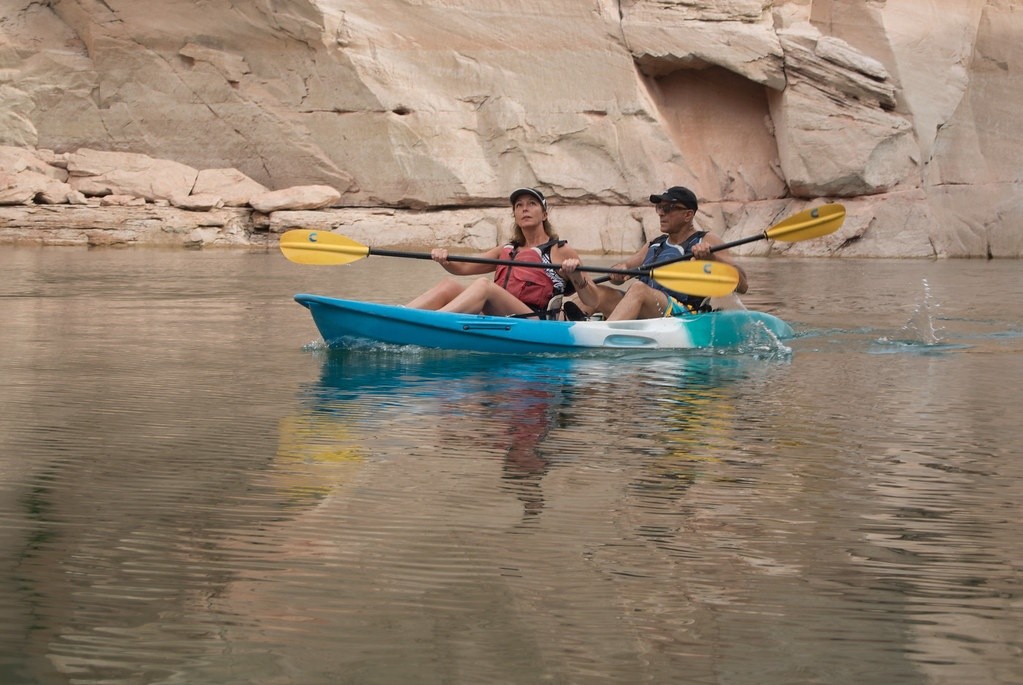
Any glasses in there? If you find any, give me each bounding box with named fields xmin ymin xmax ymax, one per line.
xmin=656 ymin=204 xmax=689 ymax=215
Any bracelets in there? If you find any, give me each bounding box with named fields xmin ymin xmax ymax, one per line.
xmin=574 ymin=277 xmax=588 ymax=289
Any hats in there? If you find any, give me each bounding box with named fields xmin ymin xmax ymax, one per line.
xmin=510 ymin=188 xmax=547 ymax=211
xmin=650 ymin=186 xmax=698 ymax=214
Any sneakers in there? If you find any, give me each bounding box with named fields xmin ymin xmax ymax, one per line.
xmin=564 ymin=301 xmax=591 ymax=321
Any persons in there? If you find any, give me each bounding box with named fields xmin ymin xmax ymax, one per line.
xmin=395 ymin=185 xmax=599 ymax=321
xmin=553 ymin=185 xmax=745 ymax=321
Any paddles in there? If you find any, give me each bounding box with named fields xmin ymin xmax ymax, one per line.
xmin=278 ymin=229 xmax=738 ymax=297
xmin=593 ymin=203 xmax=846 ymax=283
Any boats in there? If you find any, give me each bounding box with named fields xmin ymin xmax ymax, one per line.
xmin=293 ymin=293 xmax=796 ymax=352
